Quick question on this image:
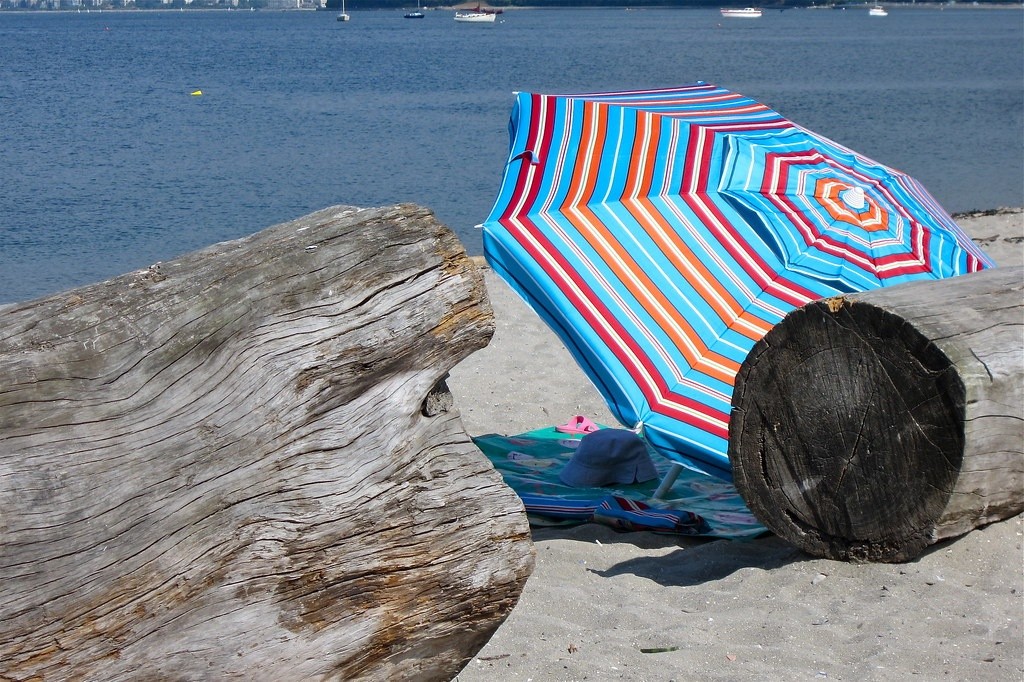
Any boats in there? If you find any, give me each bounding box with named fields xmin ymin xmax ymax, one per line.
xmin=720 ymin=7 xmax=763 ymax=19
xmin=869 ymin=5 xmax=889 ymax=18
xmin=402 ymin=9 xmax=425 ymax=19
xmin=454 ymin=11 xmax=500 ymax=23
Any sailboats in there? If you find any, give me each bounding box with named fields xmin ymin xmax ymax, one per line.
xmin=336 ymin=0 xmax=351 ymax=21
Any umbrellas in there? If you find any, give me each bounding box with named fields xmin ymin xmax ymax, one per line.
xmin=475 ymin=79 xmax=998 ymax=487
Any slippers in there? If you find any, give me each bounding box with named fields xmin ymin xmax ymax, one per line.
xmin=555 ymin=415 xmax=599 ymax=433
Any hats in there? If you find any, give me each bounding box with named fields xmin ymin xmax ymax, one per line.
xmin=560 ymin=428 xmax=658 ymax=489
